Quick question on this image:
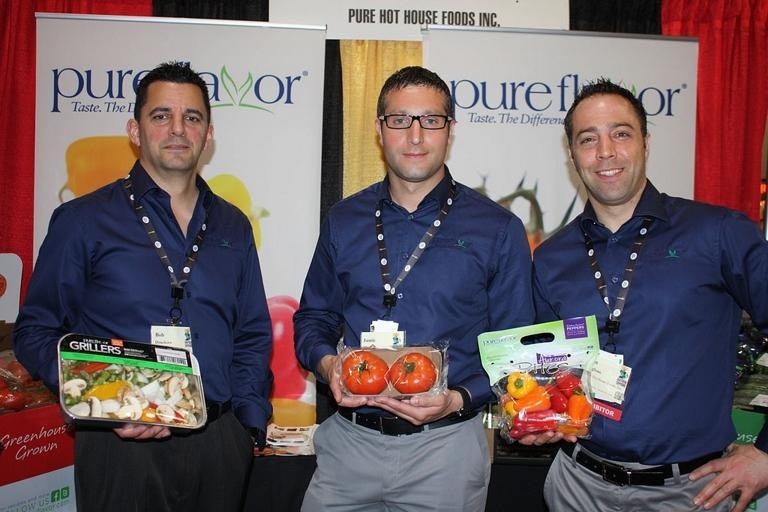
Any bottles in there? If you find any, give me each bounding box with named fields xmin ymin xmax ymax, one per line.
xmin=732 ymin=334 xmax=754 ymax=389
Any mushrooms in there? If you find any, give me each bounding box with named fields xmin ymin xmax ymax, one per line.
xmin=63 ymin=368 xmax=202 ymax=424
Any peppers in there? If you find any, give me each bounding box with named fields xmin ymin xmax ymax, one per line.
xmin=502 ymin=372 xmax=594 ymax=440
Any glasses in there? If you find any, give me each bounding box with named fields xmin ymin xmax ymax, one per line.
xmin=378 ymin=114 xmax=453 ymax=130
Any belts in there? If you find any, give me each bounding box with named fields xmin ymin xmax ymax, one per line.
xmin=207 ymin=399 xmax=233 ymax=421
xmin=337 ymin=404 xmax=479 ymax=436
xmin=559 ymin=438 xmax=727 ymax=488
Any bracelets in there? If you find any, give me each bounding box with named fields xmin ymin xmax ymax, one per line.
xmin=449 ymin=386 xmax=473 ymax=418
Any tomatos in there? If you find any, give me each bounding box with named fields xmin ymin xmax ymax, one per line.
xmin=390 ymin=354 xmax=437 ymax=394
xmin=0 ymin=354 xmax=52 ymax=411
xmin=344 ymin=351 xmax=389 ymax=395
xmin=266 ymin=295 xmax=309 ymax=400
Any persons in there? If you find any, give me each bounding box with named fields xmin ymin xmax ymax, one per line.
xmin=292 ymin=67 xmax=535 ymax=512
xmin=501 ymin=81 xmax=767 ymax=511
xmin=11 ymin=66 xmax=273 ymax=512
